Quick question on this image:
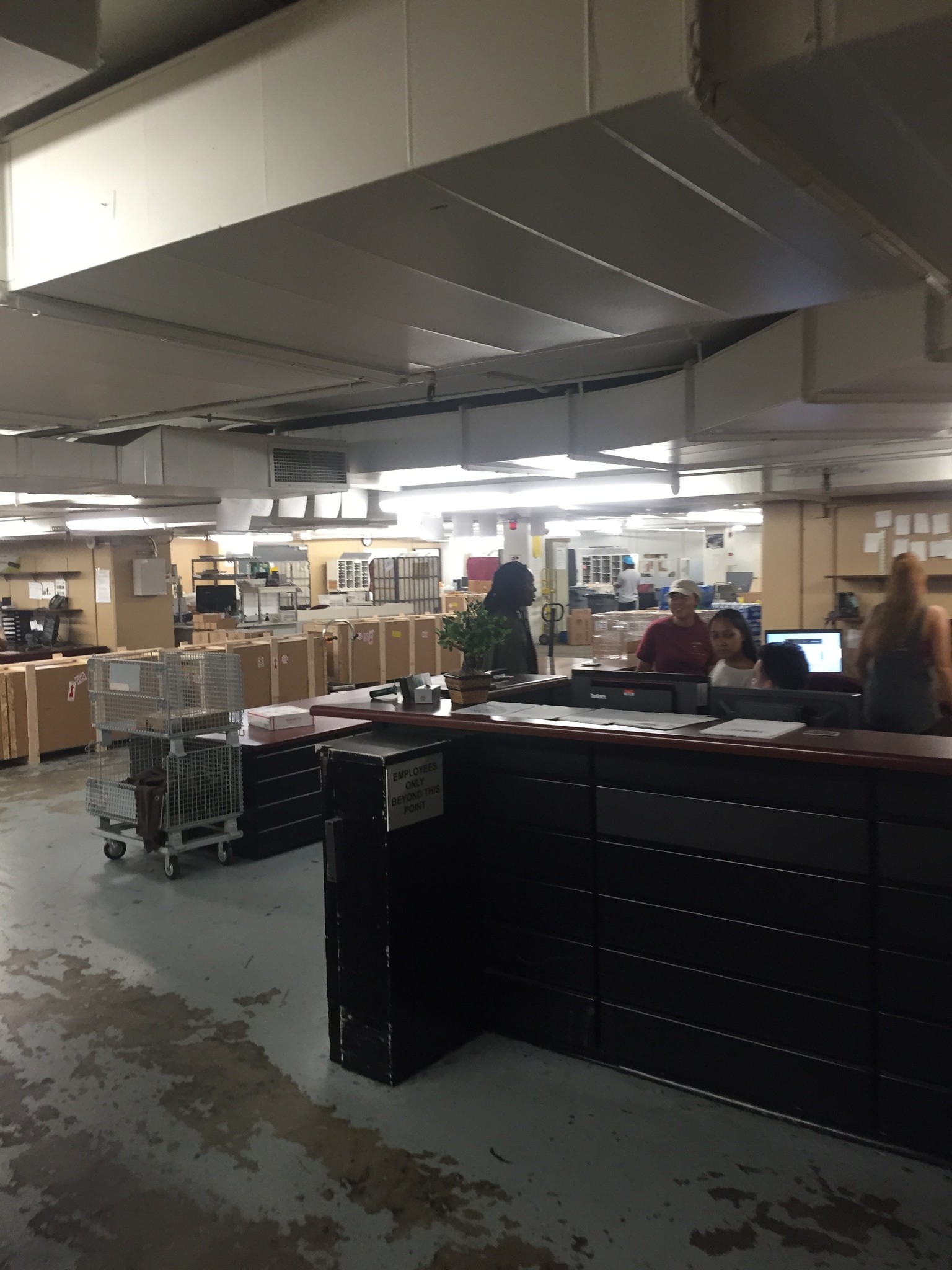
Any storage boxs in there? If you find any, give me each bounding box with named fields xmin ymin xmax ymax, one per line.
xmin=440 ymin=556 xmax=499 ymax=614
xmin=1 ymin=613 xmax=460 ymax=765
xmin=568 ymin=585 xmax=763 ymax=661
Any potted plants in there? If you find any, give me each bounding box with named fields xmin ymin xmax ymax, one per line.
xmin=435 ymin=596 xmax=512 ymax=706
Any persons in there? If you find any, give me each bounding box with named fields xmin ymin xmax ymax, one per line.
xmin=854 ymin=550 xmax=952 ymax=738
xmin=751 ymin=642 xmax=810 ymax=690
xmin=634 ymin=578 xmax=719 ymax=675
xmin=460 ymin=561 xmax=540 ymax=675
xmin=612 ymin=556 xmax=641 ymax=612
xmin=708 ymin=608 xmax=759 ymax=690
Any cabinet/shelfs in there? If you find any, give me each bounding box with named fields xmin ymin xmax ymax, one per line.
xmin=171 ymin=545 xmax=441 ymax=625
xmin=579 ymin=553 xmax=629 ymax=586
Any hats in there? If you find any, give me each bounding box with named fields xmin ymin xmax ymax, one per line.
xmin=622 ymin=556 xmax=636 ymax=564
xmin=667 ymin=578 xmax=701 ymax=598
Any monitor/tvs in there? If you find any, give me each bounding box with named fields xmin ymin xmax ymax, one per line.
xmin=569 ymin=668 xmax=710 ymax=715
xmin=39 ymin=615 xmax=60 ymax=645
xmin=764 ymin=627 xmax=844 ymax=676
xmin=195 ymin=584 xmax=237 ymax=613
xmin=708 ymin=684 xmax=866 ymax=731
